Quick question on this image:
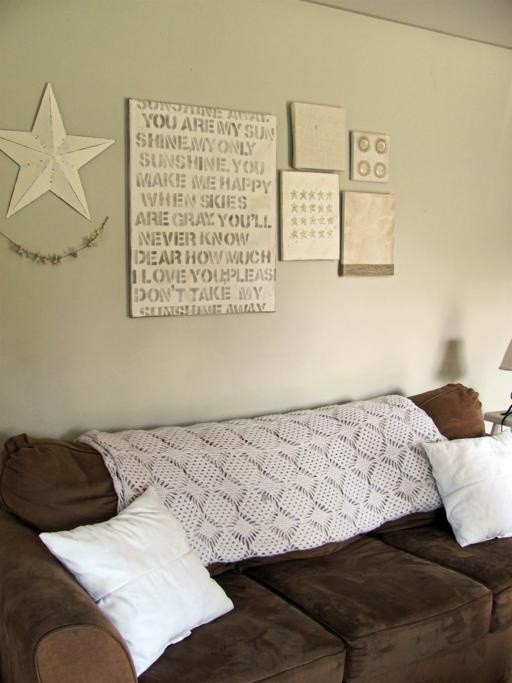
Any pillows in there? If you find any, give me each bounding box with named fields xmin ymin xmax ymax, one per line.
xmin=39 ymin=486 xmax=234 ymax=680
xmin=422 ymin=437 xmax=512 ymax=548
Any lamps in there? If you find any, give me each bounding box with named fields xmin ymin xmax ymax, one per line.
xmin=499 ymin=340 xmax=512 ymax=415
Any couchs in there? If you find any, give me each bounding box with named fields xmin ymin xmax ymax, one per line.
xmin=0 ymin=383 xmax=512 ymax=681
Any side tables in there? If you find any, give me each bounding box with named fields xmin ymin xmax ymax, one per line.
xmin=484 ymin=411 xmax=512 ymax=434
